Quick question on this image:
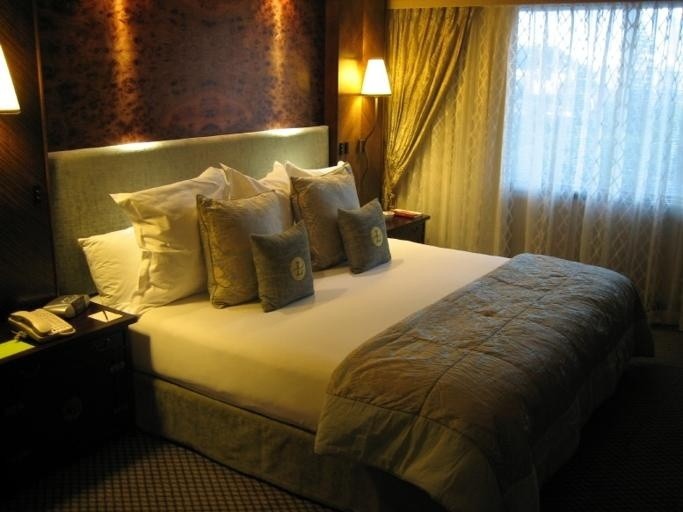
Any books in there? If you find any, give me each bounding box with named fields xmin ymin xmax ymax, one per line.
xmin=392 ymin=208 xmax=422 ymax=219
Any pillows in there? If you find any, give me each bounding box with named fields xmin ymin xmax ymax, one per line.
xmin=108 ymin=165 xmax=227 ymax=309
xmin=77 ymin=224 xmax=146 ymax=311
xmin=336 ymin=198 xmax=392 ymax=275
xmin=249 ymin=221 xmax=314 ymax=311
xmin=285 ymin=160 xmax=359 ymax=271
xmin=220 ymin=158 xmax=291 ymax=197
xmin=196 ymin=189 xmax=297 ymax=310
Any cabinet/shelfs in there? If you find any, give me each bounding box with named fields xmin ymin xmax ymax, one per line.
xmin=0 ymin=294 xmax=136 ymax=502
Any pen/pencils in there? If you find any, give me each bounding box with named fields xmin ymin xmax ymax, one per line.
xmin=102 ymin=309 xmax=108 ymax=320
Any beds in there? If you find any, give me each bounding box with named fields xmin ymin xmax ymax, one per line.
xmin=46 ymin=124 xmax=653 ymax=510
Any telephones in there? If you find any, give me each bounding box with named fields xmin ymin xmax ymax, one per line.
xmin=6 ymin=305 xmax=76 ymax=345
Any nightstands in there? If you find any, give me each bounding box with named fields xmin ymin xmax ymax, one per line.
xmin=381 ymin=211 xmax=429 ymax=244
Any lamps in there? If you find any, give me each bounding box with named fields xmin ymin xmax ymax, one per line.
xmin=359 ymin=57 xmax=392 ymax=207
